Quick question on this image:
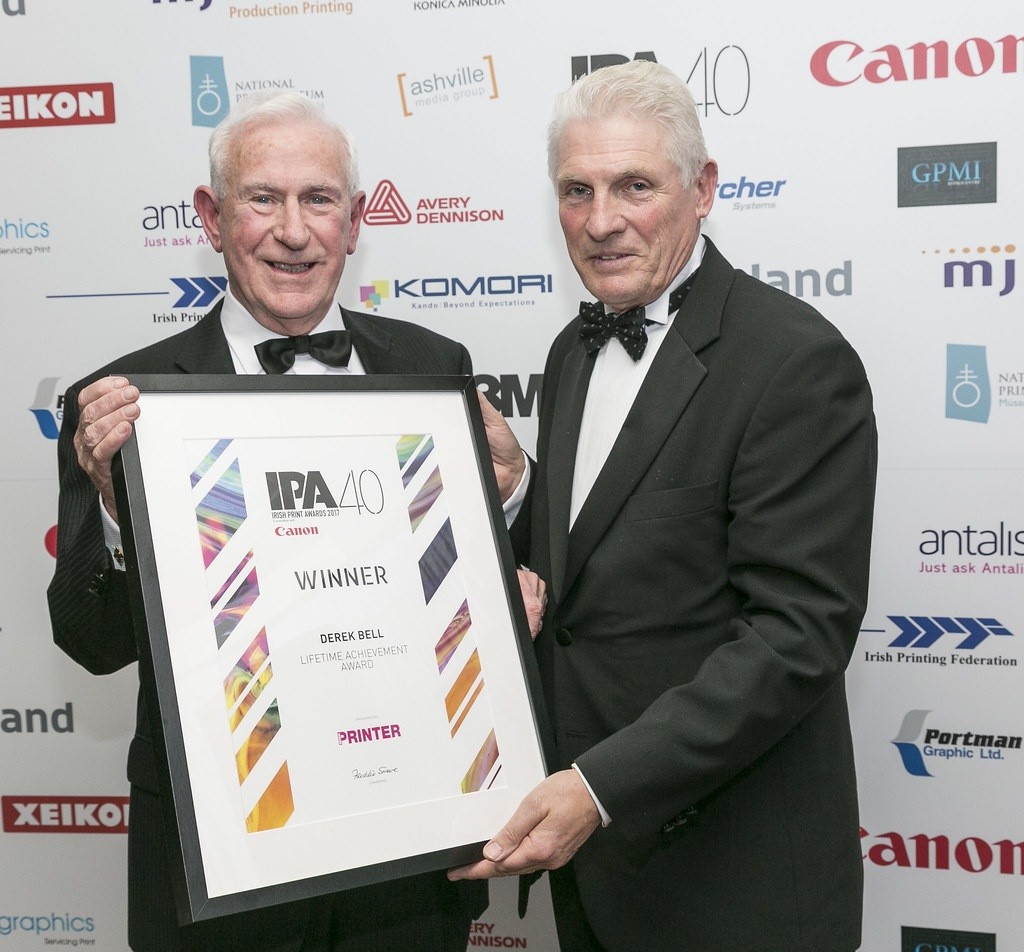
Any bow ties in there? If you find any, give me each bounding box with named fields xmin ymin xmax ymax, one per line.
xmin=579 ymin=267 xmax=705 ymax=361
xmin=253 ymin=328 xmax=352 ymax=374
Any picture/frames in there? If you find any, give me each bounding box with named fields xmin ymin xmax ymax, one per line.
xmin=105 ymin=373 xmax=559 ymax=928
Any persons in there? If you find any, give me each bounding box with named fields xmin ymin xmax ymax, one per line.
xmin=450 ymin=60 xmax=879 ymax=952
xmin=46 ymin=88 xmax=548 ymax=952
